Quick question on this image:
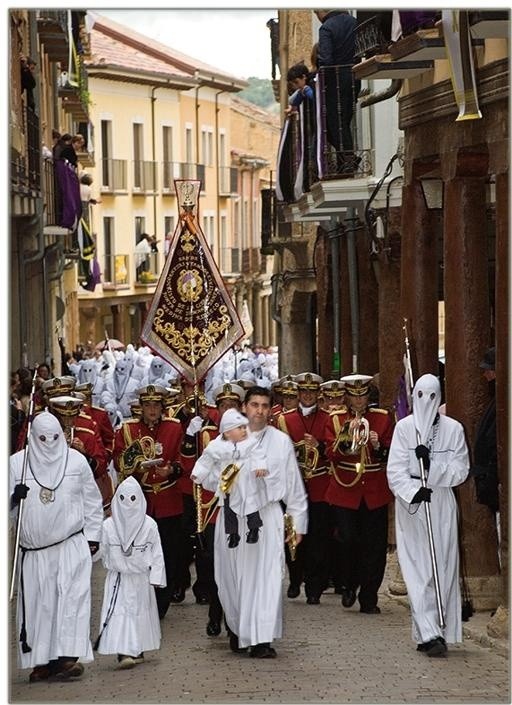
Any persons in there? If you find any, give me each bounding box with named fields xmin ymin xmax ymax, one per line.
xmin=280 ymin=8 xmax=359 ymax=190
xmin=134 ymin=230 xmax=174 ymax=283
xmin=49 ymin=129 xmax=102 ymax=229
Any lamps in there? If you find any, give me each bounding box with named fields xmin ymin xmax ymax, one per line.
xmin=417 ymin=165 xmax=447 ymax=214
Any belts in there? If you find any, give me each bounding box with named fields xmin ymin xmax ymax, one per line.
xmin=299 ymin=466 xmax=328 ymax=479
xmin=336 ymin=461 xmax=382 ymax=473
xmin=139 ymin=480 xmax=176 ymax=494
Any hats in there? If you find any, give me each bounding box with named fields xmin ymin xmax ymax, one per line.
xmin=164 ymin=373 xmax=206 ymax=406
xmin=127 ymin=399 xmax=144 ymax=415
xmin=219 ymin=408 xmax=250 ymax=434
xmin=64 ymin=375 xmax=97 ymax=401
xmin=295 ymin=372 xmax=323 ymax=391
xmin=319 ymin=380 xmax=346 ymax=398
xmin=270 ymin=373 xmax=299 ymax=397
xmin=212 ymin=383 xmax=246 ymax=403
xmin=43 ymin=377 xmax=75 ymax=394
xmin=231 ymin=379 xmax=256 ymax=392
xmin=340 ymin=374 xmax=374 ymax=396
xmin=49 ymin=396 xmax=84 ymax=417
xmin=478 ymin=346 xmax=495 ymax=370
xmin=135 ymin=384 xmax=169 ymax=401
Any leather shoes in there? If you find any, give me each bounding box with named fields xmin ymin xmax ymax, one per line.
xmin=29 ymin=665 xmax=53 ymax=683
xmin=342 ymin=588 xmax=356 ymax=608
xmin=196 ymin=594 xmax=209 ymax=605
xmin=172 ymin=587 xmax=186 ymax=603
xmin=207 ymin=620 xmax=221 ymax=636
xmin=287 ymin=584 xmax=300 ymax=599
xmin=359 ymin=606 xmax=381 ymax=615
xmin=307 ymin=598 xmax=320 ymax=605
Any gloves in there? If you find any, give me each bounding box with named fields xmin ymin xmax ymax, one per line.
xmin=411 ymin=487 xmax=433 ymax=505
xmin=86 ymin=539 xmax=100 ymax=556
xmin=185 ymin=416 xmax=204 ymax=437
xmin=415 ymin=444 xmax=430 ymax=472
xmin=7 ymin=484 xmax=30 ymax=511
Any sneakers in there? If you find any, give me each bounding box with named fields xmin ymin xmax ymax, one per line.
xmin=225 ymin=625 xmax=248 ymax=653
xmin=251 ymin=649 xmax=277 ymax=659
xmin=417 ymin=638 xmax=448 ymax=658
xmin=54 ymin=662 xmax=85 ymax=682
xmin=118 ymin=657 xmax=135 ymax=670
xmin=135 ymin=659 xmax=145 ymax=665
xmin=246 ymin=529 xmax=259 ymax=544
xmin=334 ymin=154 xmax=362 ymax=179
xmin=228 ymin=534 xmax=241 ymax=549
